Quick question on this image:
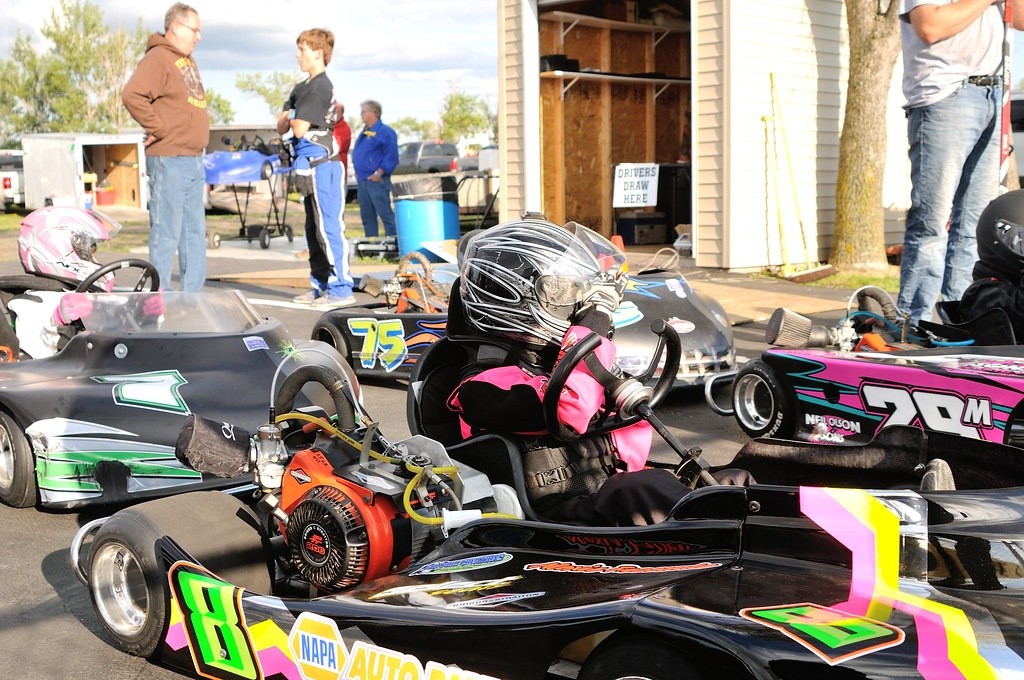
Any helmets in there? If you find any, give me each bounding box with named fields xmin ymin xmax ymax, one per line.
xmin=461 ymin=220 xmax=598 ymax=351
xmin=18 ymin=205 xmax=115 ymax=292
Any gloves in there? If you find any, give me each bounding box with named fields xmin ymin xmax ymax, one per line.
xmin=574 ymin=262 xmax=629 ymax=316
xmin=52 ymin=291 xmax=93 ymax=326
xmin=137 ymin=288 xmax=164 ymax=316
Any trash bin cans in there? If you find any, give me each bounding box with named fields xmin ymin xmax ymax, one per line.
xmin=389 ymin=174 xmax=462 ymax=265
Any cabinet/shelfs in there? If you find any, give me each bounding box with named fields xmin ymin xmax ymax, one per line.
xmin=539 ymin=11 xmax=691 ymax=85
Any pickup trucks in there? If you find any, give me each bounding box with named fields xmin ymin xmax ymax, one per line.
xmin=388 ymin=140 xmax=480 ymax=175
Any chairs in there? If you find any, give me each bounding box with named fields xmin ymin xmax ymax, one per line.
xmin=407 ymin=275 xmax=545 ymax=522
xmin=936 ymin=300 xmax=1017 ymax=346
xmin=0 ymin=274 xmax=28 ymax=363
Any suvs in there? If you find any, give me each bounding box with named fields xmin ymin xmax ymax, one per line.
xmin=0 ymin=147 xmax=25 ymax=211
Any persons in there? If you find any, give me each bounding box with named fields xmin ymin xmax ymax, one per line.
xmin=445 ymin=220 xmax=757 ymax=526
xmin=7 ymin=205 xmax=163 ymax=360
xmin=122 ymin=3 xmax=210 ymax=303
xmin=277 ymin=28 xmax=357 ymax=312
xmin=351 ymin=100 xmax=400 ymax=237
xmin=897 ymin=0 xmax=1024 ymax=343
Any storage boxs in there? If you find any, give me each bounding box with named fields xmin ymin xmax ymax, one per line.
xmin=619 ymin=210 xmax=669 ymax=246
xmin=96 ymin=187 xmax=113 ymax=205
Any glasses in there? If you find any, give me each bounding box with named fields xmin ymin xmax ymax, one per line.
xmin=176 ymin=22 xmax=200 ymax=33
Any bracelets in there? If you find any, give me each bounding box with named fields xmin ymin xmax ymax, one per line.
xmin=374 ymin=171 xmax=382 ymax=177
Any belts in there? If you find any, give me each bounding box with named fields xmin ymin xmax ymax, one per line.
xmin=969 ymin=76 xmax=1000 ymax=86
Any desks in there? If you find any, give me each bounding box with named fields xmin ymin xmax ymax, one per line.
xmin=612 ymin=162 xmax=691 ymax=242
xmin=84 ymin=173 xmax=98 ymax=206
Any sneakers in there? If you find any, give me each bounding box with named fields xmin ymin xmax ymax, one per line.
xmin=312 ymin=288 xmax=355 ymax=308
xmin=293 ymin=290 xmax=326 ymax=304
